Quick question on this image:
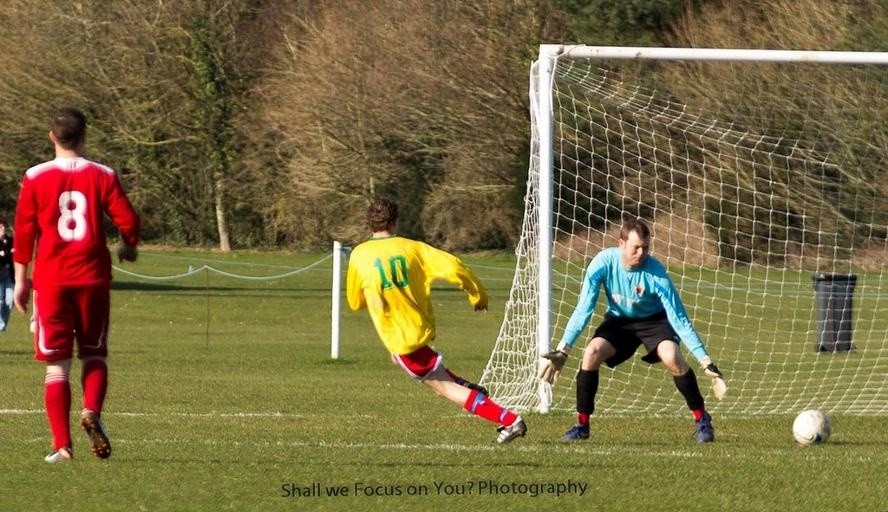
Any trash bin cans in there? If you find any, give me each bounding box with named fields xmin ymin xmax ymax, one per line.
xmin=815 ymin=275 xmax=855 ymax=352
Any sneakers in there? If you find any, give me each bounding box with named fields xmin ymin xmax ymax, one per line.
xmin=694 ymin=411 xmax=714 ymax=444
xmin=79 ymin=408 xmax=110 ymax=458
xmin=45 ymin=446 xmax=73 ymax=464
xmin=560 ymin=423 xmax=589 ymax=439
xmin=496 ymin=415 xmax=527 ymax=445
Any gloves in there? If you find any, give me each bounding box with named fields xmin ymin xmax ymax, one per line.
xmin=703 ymin=363 xmax=727 ymax=400
xmin=538 ymin=350 xmax=567 ymax=386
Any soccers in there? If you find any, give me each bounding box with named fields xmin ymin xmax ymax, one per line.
xmin=792 ymin=409 xmax=830 ymax=445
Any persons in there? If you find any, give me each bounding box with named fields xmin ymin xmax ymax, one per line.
xmin=0 ymin=220 xmax=15 ymax=331
xmin=538 ymin=219 xmax=729 ymax=444
xmin=14 ymin=110 xmax=142 ymax=463
xmin=345 ymin=199 xmax=529 ymax=444
